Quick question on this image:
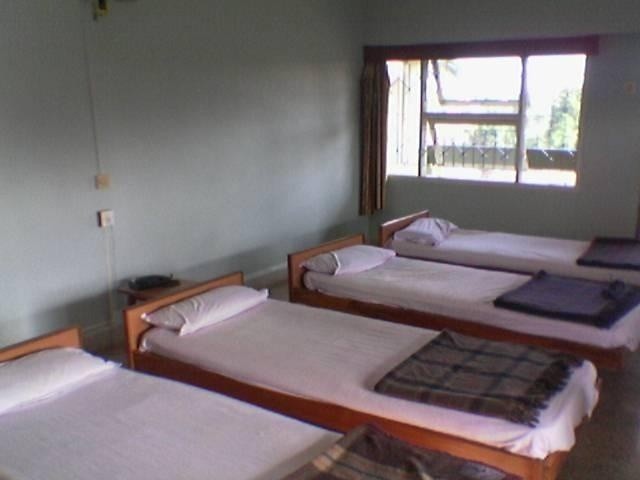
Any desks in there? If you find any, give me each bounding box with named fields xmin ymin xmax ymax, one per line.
xmin=116 ymin=276 xmax=197 ymax=307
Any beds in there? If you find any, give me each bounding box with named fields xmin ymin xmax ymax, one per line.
xmin=121 ymin=269 xmax=602 ymax=479
xmin=286 ymin=230 xmax=639 ymax=373
xmin=377 ymin=208 xmax=639 ymax=285
xmin=0 ymin=326 xmax=530 ymax=480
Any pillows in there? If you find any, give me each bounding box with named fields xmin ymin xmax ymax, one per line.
xmin=393 ymin=216 xmax=458 ymax=246
xmin=298 ymin=242 xmax=398 ymax=277
xmin=1 ymin=344 xmax=123 ymax=416
xmin=141 ymin=283 xmax=271 ymax=338
